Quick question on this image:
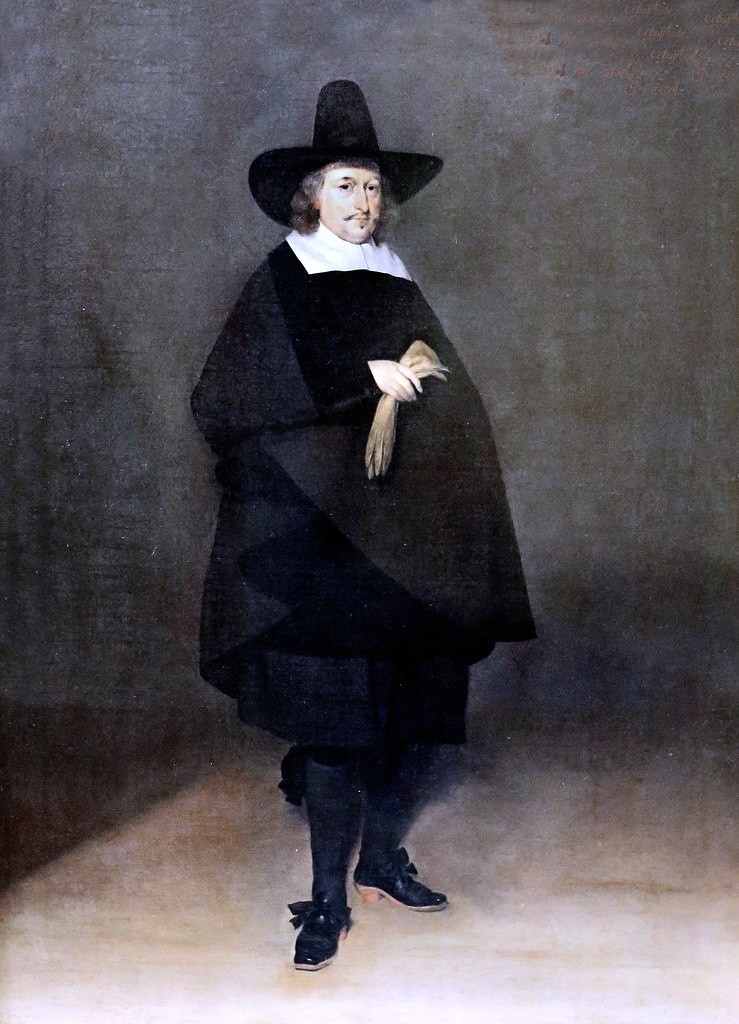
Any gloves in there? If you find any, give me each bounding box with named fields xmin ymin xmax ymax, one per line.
xmin=365 ymin=341 xmax=450 ymax=480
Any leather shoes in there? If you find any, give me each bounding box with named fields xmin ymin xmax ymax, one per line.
xmin=354 ymin=848 xmax=447 ymax=911
xmin=288 ymin=885 xmax=353 ymax=970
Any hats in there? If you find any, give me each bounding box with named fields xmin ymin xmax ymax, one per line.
xmin=248 ymin=80 xmax=445 ymax=229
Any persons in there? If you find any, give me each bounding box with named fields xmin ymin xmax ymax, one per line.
xmin=192 ymin=78 xmax=538 ymax=971
